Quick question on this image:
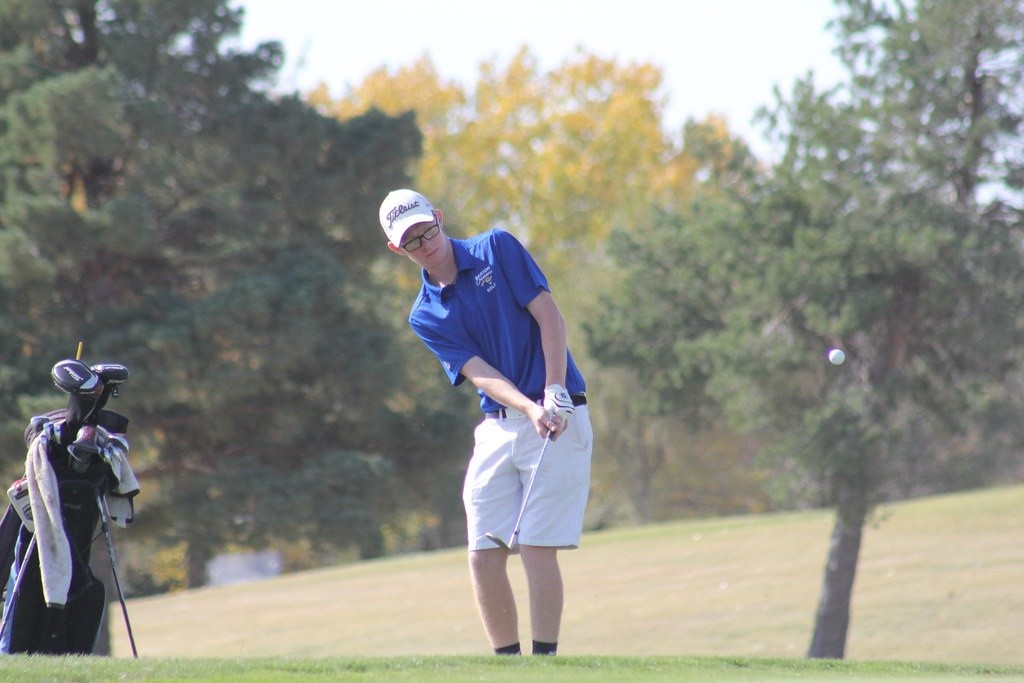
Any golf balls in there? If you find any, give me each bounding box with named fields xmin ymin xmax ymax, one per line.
xmin=828 ymin=348 xmax=844 ymax=364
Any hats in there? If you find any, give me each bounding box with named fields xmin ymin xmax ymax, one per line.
xmin=379 ymin=189 xmax=434 ymax=248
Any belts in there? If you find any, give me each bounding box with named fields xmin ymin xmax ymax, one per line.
xmin=486 ymin=395 xmax=587 ymax=419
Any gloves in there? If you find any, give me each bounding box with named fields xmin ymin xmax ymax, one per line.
xmin=543 ymin=384 xmax=575 ymax=428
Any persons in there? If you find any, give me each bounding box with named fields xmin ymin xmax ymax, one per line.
xmin=380 ymin=189 xmax=593 ymax=656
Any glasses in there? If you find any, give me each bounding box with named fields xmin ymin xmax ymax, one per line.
xmin=401 ymin=215 xmax=440 ymax=252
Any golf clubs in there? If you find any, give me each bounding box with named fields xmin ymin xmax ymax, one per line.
xmin=96 ymin=434 xmax=130 ymax=463
xmin=481 ymin=429 xmax=554 ymax=554
